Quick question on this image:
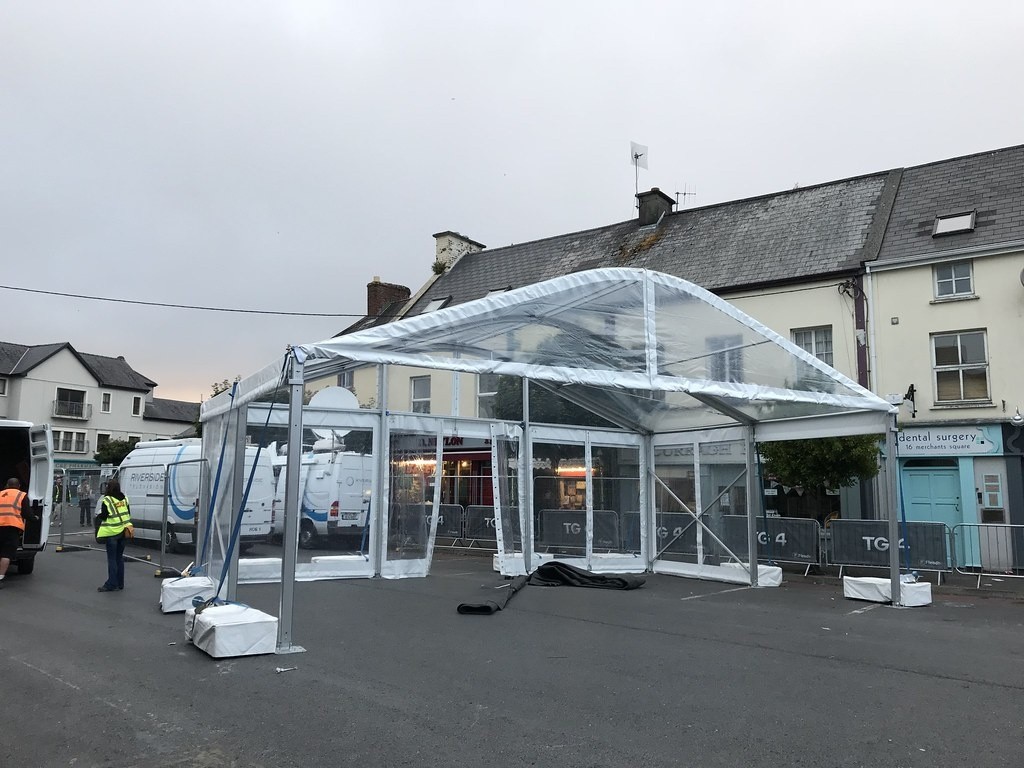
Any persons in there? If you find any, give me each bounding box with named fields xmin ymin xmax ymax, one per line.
xmin=50 ymin=477 xmax=72 ymax=527
xmin=95 ymin=480 xmax=133 ymax=592
xmin=0 ymin=478 xmax=39 ymax=584
xmin=77 ymin=479 xmax=92 ymax=527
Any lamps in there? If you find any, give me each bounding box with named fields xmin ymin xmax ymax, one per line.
xmin=1009 ymin=406 xmax=1024 ymax=427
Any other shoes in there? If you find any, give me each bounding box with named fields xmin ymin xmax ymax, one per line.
xmin=50 ymin=522 xmax=62 ymax=527
xmin=97 ymin=583 xmax=124 ymax=593
xmin=80 ymin=523 xmax=93 ymax=528
xmin=0 ymin=575 xmax=7 ymax=583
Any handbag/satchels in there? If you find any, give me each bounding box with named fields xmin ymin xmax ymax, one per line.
xmin=125 ymin=525 xmax=135 ymax=538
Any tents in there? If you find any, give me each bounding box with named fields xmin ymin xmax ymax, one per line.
xmin=191 ymin=266 xmax=900 ymax=606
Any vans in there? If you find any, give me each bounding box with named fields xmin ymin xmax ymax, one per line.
xmin=0 ymin=418 xmax=54 ymax=575
xmin=265 ymin=441 xmax=373 ymax=549
xmin=93 ymin=438 xmax=276 ymax=554
